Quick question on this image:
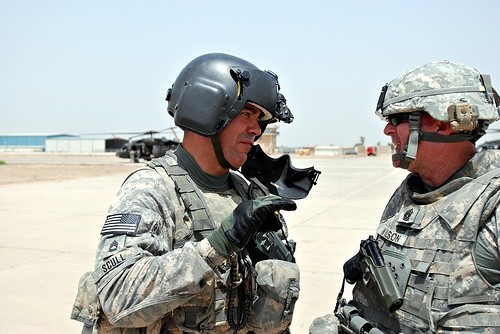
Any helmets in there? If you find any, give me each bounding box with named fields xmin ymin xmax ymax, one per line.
xmin=375 ymin=61 xmax=500 ymax=131
xmin=165 ymin=53 xmax=294 ymax=137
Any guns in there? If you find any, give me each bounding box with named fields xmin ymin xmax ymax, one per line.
xmin=343 ymin=234 xmax=405 ymax=314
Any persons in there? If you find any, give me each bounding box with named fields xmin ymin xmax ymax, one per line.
xmin=70 ymin=53 xmax=300 ymax=334
xmin=333 ymin=61 xmax=500 ymax=334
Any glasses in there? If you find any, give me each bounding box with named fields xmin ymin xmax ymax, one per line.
xmin=388 ymin=116 xmax=409 ymax=126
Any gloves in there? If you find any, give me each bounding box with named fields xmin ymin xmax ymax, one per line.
xmin=207 ymin=195 xmax=297 ymax=259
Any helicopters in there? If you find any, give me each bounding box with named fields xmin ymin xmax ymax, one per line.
xmin=116 ymin=126 xmax=181 ymax=162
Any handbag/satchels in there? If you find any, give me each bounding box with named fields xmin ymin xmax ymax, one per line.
xmin=245 ymin=260 xmax=300 ymax=334
